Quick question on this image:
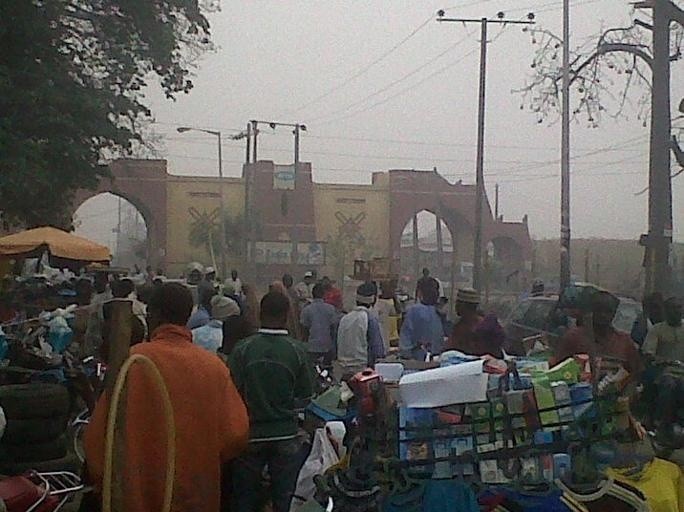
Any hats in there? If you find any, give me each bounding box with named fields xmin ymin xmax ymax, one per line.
xmin=211 ymin=296 xmax=240 ymax=322
xmin=355 ymin=283 xmax=377 ymax=304
xmin=206 ymin=266 xmax=214 ymax=274
xmin=304 ymin=271 xmax=313 ymax=278
xmin=457 ymin=288 xmax=479 ymax=304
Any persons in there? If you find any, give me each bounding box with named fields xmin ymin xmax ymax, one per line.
xmin=0 ymin=265 xmax=683 ymax=512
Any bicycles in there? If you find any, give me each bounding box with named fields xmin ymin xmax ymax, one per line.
xmin=69 ymin=355 xmax=108 ymax=464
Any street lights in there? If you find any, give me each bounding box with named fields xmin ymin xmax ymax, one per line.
xmin=176 ymin=124 xmax=228 ymax=279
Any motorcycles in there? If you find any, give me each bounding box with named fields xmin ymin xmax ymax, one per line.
xmin=0 ymin=468 xmax=83 ymax=512
xmin=644 ymin=355 xmax=683 ymax=462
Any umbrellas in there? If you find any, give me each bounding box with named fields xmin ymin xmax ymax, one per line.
xmin=0 ymin=226 xmax=113 ymax=273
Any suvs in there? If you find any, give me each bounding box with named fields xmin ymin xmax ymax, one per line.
xmin=501 ymin=289 xmax=645 ymax=375
